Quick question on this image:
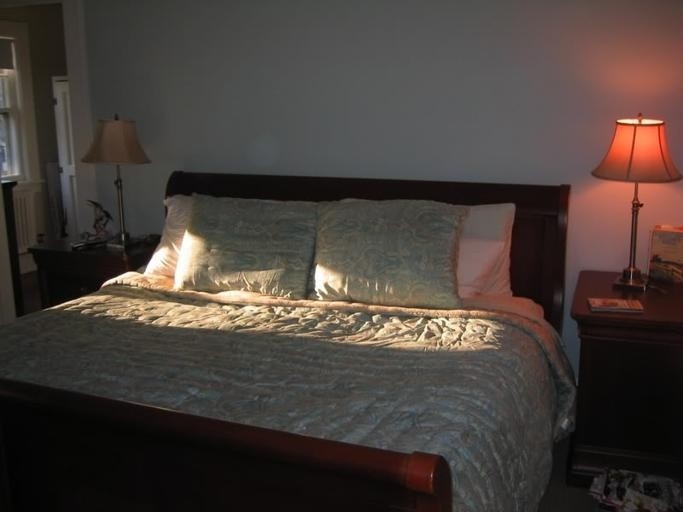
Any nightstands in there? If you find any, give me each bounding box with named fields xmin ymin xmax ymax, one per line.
xmin=566 ymin=269 xmax=682 ymax=486
xmin=28 ymin=232 xmax=150 ymax=307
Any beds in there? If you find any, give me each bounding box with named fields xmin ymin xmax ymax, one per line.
xmin=1 ymin=170 xmax=573 ymax=511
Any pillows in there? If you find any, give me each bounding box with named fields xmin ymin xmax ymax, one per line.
xmin=456 ymin=203 xmax=516 ymax=308
xmin=174 ymin=195 xmax=319 ymax=301
xmin=307 ymin=198 xmax=467 ymax=308
xmin=139 ymin=194 xmax=193 ymax=285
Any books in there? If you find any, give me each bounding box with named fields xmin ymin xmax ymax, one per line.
xmin=649 ymin=225 xmax=683 ymax=285
xmin=587 ymin=296 xmax=644 ymax=313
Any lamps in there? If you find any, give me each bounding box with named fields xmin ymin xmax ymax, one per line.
xmin=587 ymin=111 xmax=683 ymax=293
xmin=81 ymin=110 xmax=152 ymax=248
